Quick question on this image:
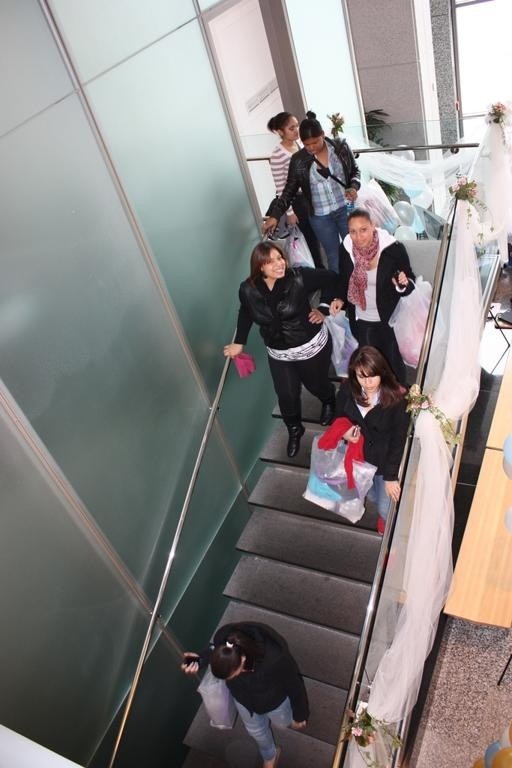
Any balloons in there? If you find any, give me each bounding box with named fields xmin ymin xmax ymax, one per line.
xmin=401 ymin=172 xmax=426 ymax=199
xmin=469 ymin=721 xmax=512 ymax=768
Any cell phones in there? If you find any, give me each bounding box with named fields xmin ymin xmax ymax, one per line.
xmin=393 ymin=270 xmax=406 ymax=290
xmin=354 ymin=427 xmax=358 ymax=437
xmin=184 ymin=656 xmax=200 ymax=668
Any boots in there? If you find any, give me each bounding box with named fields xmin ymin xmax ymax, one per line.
xmin=278 ymin=399 xmax=306 ymax=458
xmin=306 ymin=379 xmax=337 ymax=426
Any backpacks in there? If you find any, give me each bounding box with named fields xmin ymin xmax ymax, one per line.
xmin=283 ymin=223 xmax=316 ymax=270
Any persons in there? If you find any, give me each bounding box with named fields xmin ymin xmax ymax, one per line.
xmin=179 ymin=620 xmax=309 ymax=768
xmin=222 ymin=111 xmax=417 ymax=537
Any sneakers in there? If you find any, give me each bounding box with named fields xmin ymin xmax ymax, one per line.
xmin=262 ymin=745 xmax=281 ymax=768
xmin=377 ymin=515 xmax=385 ymax=535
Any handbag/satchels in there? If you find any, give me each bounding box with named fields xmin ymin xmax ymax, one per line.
xmin=303 ymin=433 xmax=378 ymax=525
xmin=319 ymin=303 xmax=362 ymax=379
xmin=349 ymin=175 xmax=400 ymax=234
xmin=390 ymin=273 xmax=435 ymax=370
xmin=196 ymin=664 xmax=239 ymax=730
